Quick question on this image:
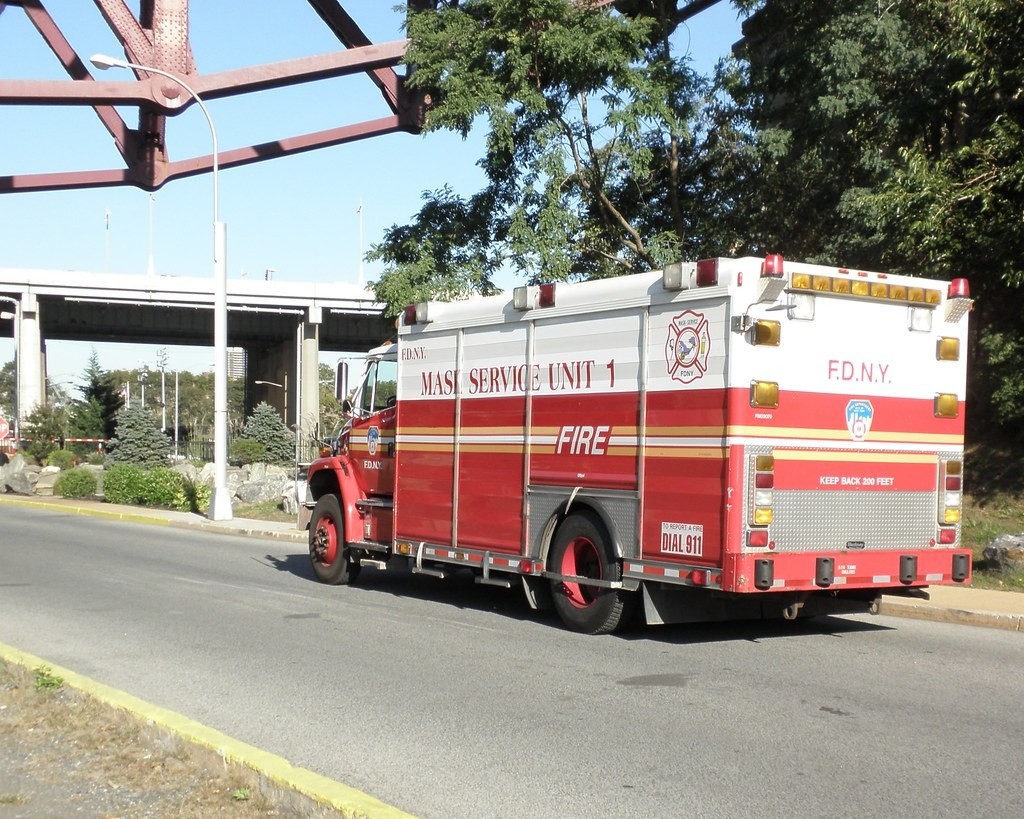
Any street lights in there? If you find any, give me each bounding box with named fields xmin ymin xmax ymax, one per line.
xmin=255 ymin=370 xmax=287 ymax=459
xmin=89 ymin=53 xmax=233 ymax=521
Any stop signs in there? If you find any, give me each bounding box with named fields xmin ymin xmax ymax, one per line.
xmin=0 ymin=416 xmax=9 ymax=440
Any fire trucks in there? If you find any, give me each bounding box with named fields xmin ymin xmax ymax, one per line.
xmin=294 ymin=255 xmax=973 ymax=635
